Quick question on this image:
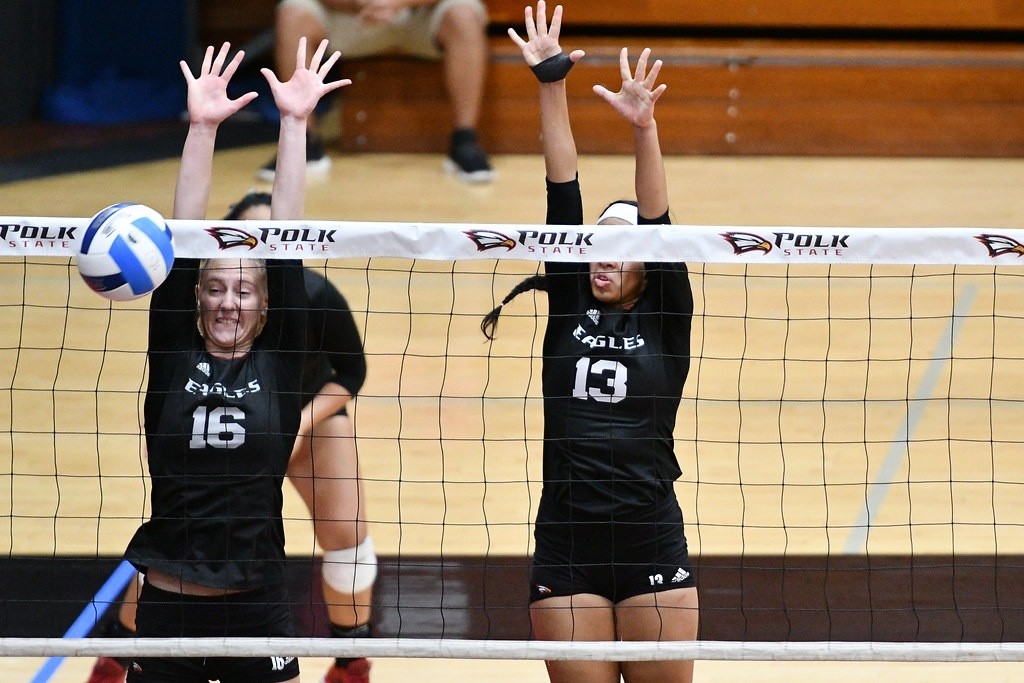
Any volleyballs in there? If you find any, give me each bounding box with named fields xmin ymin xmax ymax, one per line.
xmin=74 ymin=202 xmax=178 ymax=300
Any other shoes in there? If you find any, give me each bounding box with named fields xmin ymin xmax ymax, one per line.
xmin=256 ymin=129 xmax=331 ymax=181
xmin=442 ymin=127 xmax=493 ymax=184
xmin=87 ymin=657 xmax=128 ymax=683
xmin=324 ymin=658 xmax=370 ymax=683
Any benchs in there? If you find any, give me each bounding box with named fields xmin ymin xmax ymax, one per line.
xmin=339 ymin=0 xmax=1024 ymax=160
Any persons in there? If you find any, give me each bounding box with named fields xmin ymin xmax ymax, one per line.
xmin=84 ymin=36 xmax=379 ymax=683
xmin=257 ymin=1 xmax=496 ymax=183
xmin=481 ymin=1 xmax=700 ymax=683
